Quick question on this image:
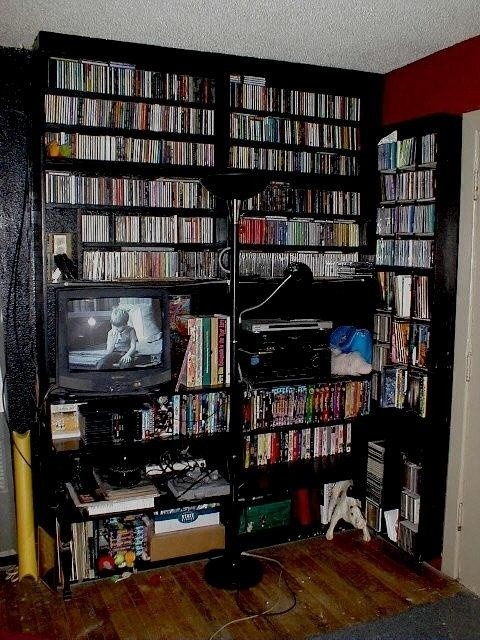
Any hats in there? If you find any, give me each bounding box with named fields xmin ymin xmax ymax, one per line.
xmin=109 ymin=309 xmax=129 ymax=325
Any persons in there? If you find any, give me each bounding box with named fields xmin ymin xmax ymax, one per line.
xmin=94 ymin=307 xmax=139 ymax=370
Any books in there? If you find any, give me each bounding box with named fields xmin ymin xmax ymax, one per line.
xmin=228 ymin=74 xmax=362 ymax=176
xmin=45 ymin=169 xmax=230 ymax=280
xmin=43 ymin=56 xmax=214 ymax=167
xmin=232 ymin=181 xmax=360 ymax=278
xmin=359 ymin=127 xmax=439 ymax=560
xmin=318 ymin=479 xmax=352 ymax=527
xmin=105 ymin=381 xmax=371 ymax=472
xmin=62 ymin=464 xmax=161 ymax=584
xmin=165 ymin=290 xmax=234 ymax=390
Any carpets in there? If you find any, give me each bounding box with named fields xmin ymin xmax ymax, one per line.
xmin=299 ymin=590 xmax=479 ymax=639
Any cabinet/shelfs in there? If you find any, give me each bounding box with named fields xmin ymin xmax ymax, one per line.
xmin=25 ymin=30 xmax=384 ymax=590
xmin=359 ymin=110 xmax=460 ymax=561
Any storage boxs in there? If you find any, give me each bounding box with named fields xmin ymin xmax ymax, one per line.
xmin=149 ymin=501 xmax=220 ymax=534
xmin=146 ymin=522 xmax=224 ymax=563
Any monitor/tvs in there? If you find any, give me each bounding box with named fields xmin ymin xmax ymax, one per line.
xmin=55 ymin=286 xmax=173 ymax=396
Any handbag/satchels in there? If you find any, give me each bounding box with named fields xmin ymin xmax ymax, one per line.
xmin=329 ymin=325 xmax=374 ymax=377
